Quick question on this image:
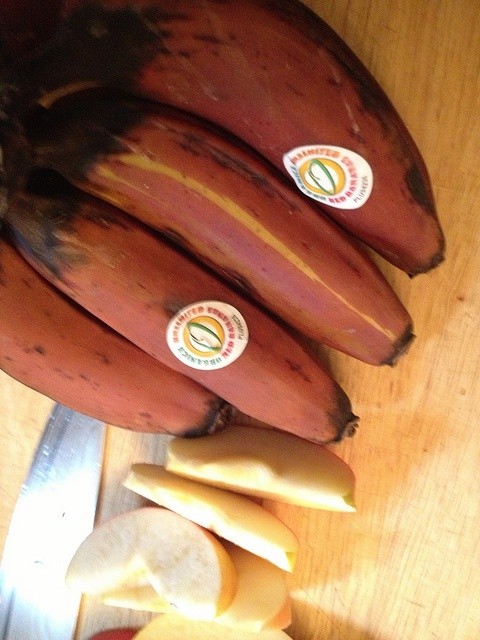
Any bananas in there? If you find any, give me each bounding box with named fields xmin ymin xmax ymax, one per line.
xmin=0 ymin=2 xmax=444 ymax=443
